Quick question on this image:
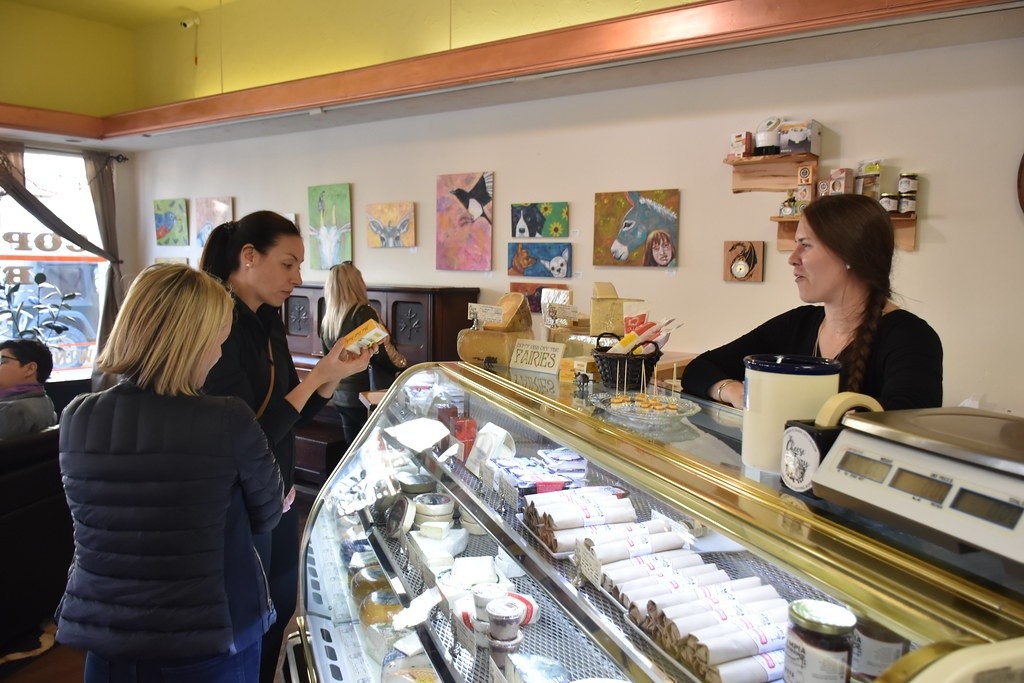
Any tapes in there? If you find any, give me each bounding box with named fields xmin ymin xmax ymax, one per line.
xmin=458 ymin=505 xmax=487 ymax=536
xmin=413 ymin=493 xmax=455 ymax=530
xmin=815 ymin=392 xmax=884 ymax=430
xmin=386 ymin=495 xmax=417 ymax=538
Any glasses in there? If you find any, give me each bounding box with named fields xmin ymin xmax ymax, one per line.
xmin=329 ymin=261 xmax=352 ymax=271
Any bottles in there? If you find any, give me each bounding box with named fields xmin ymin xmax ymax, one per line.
xmin=783 ymin=599 xmax=857 ymax=683
xmin=847 ymin=606 xmax=910 ymax=683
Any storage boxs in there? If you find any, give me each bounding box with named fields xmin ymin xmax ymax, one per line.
xmin=729 ymin=132 xmax=751 ymax=157
xmin=780 ymin=118 xmax=822 ymax=157
xmin=545 ymin=279 xmax=648 ymax=361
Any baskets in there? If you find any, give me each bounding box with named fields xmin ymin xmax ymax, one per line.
xmin=591 ymin=333 xmax=663 ymax=391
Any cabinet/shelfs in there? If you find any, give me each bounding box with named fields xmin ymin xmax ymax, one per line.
xmin=272 ymin=282 xmax=480 ymax=510
xmin=275 ymin=358 xmax=1024 ymax=683
xmin=725 ymin=152 xmax=917 ymax=253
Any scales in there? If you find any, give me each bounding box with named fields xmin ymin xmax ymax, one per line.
xmin=811 ymin=406 xmax=1024 ymax=604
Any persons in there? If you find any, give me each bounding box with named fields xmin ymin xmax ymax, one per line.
xmin=319 ymin=260 xmax=406 ymax=445
xmin=682 ymin=194 xmax=944 ymax=412
xmin=200 ymin=211 xmax=379 ymax=683
xmin=0 ymin=338 xmax=58 ymax=438
xmin=52 ymin=262 xmax=285 ymax=683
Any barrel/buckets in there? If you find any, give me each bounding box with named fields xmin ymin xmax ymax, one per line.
xmin=741 ymin=355 xmax=842 ymax=475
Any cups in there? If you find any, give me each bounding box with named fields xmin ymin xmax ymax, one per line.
xmin=780 ymin=419 xmax=846 ymax=500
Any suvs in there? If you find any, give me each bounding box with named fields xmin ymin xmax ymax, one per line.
xmin=0 ymin=261 xmax=100 ymax=369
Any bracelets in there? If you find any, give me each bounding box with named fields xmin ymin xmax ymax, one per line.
xmin=717 ymin=380 xmax=738 ymax=402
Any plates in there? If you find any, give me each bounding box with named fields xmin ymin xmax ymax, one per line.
xmin=588 ymin=392 xmax=702 ymax=424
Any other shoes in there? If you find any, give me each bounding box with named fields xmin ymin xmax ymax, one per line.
xmin=0 ymin=631 xmax=54 ymax=667
